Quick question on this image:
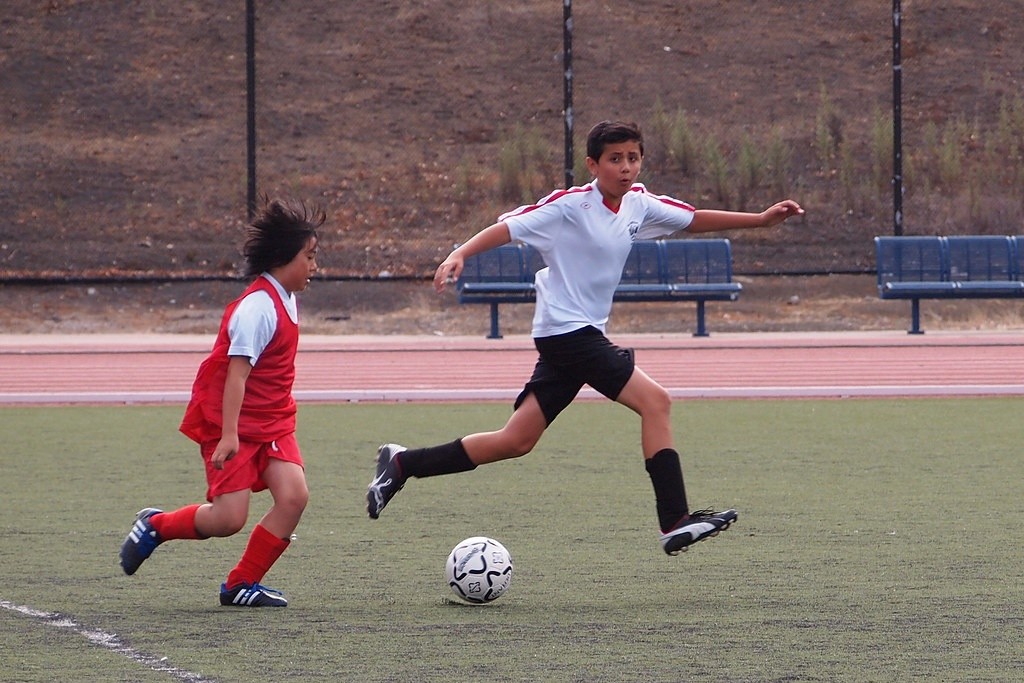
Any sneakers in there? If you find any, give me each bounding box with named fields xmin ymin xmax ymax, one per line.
xmin=118 ymin=507 xmax=164 ymax=576
xmin=219 ymin=580 xmax=288 ymax=607
xmin=657 ymin=505 xmax=738 ymax=556
xmin=365 ymin=443 xmax=408 ymax=520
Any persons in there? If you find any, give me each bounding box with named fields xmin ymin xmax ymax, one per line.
xmin=121 ymin=195 xmax=326 ymax=606
xmin=366 ymin=119 xmax=806 ymax=556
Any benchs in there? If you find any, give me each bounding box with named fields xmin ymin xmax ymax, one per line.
xmin=872 ymin=236 xmax=1024 ymax=334
xmin=453 ymin=239 xmax=743 ymax=337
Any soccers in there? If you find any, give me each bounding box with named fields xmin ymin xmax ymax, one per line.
xmin=443 ymin=534 xmax=515 ymax=606
